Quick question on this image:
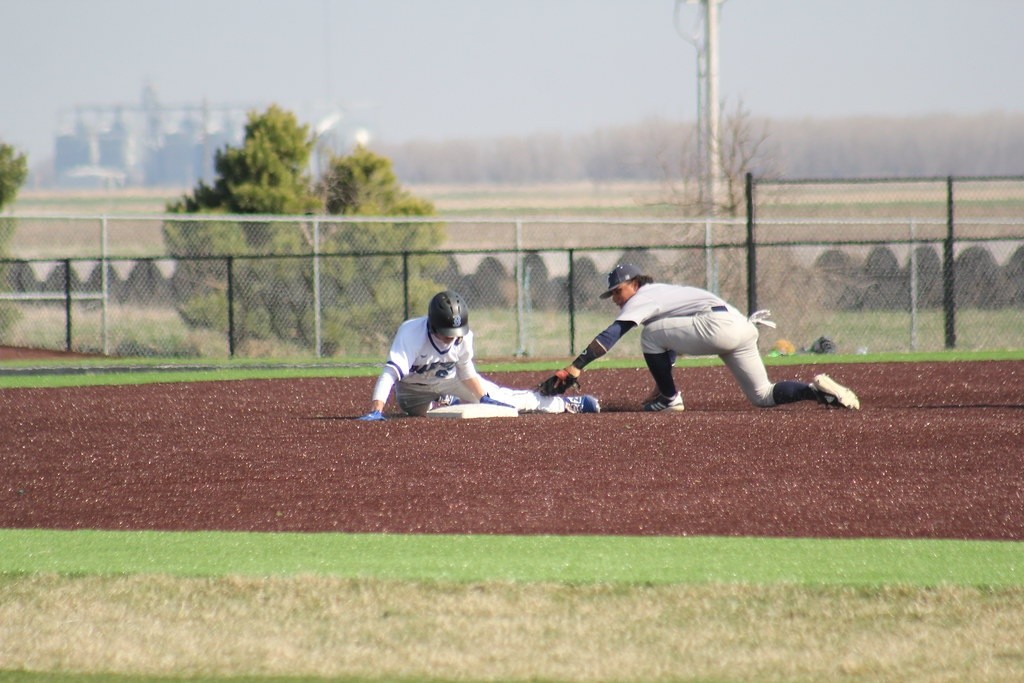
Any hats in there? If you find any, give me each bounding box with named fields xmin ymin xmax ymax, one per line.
xmin=598 ymin=263 xmax=641 ymax=299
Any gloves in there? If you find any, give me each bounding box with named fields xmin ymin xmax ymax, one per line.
xmin=353 ymin=410 xmax=387 ymax=422
xmin=479 ymin=394 xmax=515 ymax=410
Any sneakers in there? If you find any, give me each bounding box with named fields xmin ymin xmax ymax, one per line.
xmin=641 ymin=390 xmax=685 ymax=412
xmin=561 ymin=394 xmax=601 ymax=415
xmin=434 ymin=394 xmax=461 ymax=407
xmin=808 ymin=372 xmax=862 ymax=412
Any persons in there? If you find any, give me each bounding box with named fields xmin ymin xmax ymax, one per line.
xmin=533 ymin=261 xmax=860 ymax=412
xmin=353 ymin=288 xmax=601 ymax=422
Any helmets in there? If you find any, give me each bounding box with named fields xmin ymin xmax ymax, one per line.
xmin=428 ymin=291 xmax=471 ymax=338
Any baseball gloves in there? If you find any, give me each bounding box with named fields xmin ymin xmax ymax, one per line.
xmin=532 ymin=369 xmax=581 ymax=396
